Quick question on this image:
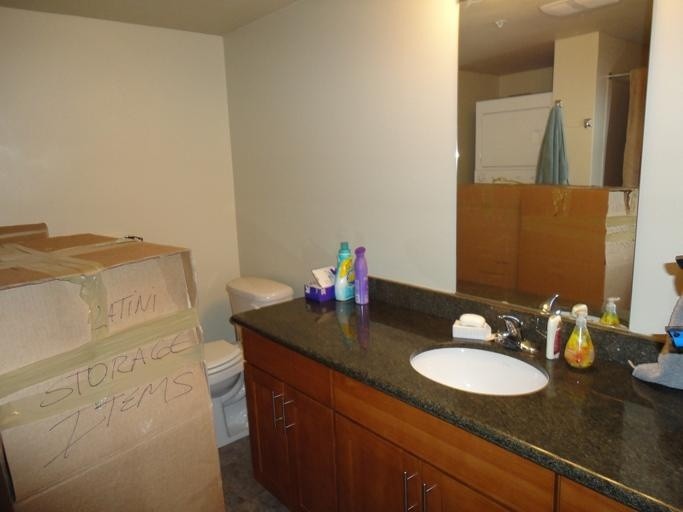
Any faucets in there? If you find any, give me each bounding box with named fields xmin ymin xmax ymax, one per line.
xmin=497 ymin=314 xmax=525 ymax=340
xmin=538 ymin=291 xmax=560 ymax=312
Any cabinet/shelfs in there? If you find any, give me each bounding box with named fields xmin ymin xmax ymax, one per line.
xmin=550 ymin=33 xmax=648 ymax=185
xmin=473 ymin=91 xmax=553 ymax=182
xmin=238 ymin=327 xmax=335 ymax=511
xmin=330 ymin=370 xmax=558 ymax=512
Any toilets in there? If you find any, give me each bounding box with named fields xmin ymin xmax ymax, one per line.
xmin=200 ymin=277 xmax=295 ymax=449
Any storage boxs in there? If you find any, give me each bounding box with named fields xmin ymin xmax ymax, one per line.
xmin=1 ymin=219 xmax=230 ymax=512
xmin=456 ymin=181 xmax=639 ymax=329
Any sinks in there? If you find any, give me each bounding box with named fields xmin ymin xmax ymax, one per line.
xmin=409 ymin=343 xmax=550 ymax=397
xmin=552 ymin=308 xmax=628 ymax=332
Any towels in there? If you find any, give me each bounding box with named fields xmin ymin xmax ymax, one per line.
xmin=620 ymin=66 xmax=648 ymax=186
xmin=532 ymin=100 xmax=571 ymax=185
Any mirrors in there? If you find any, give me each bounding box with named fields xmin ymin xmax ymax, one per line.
xmin=455 ymin=1 xmax=653 ymax=331
